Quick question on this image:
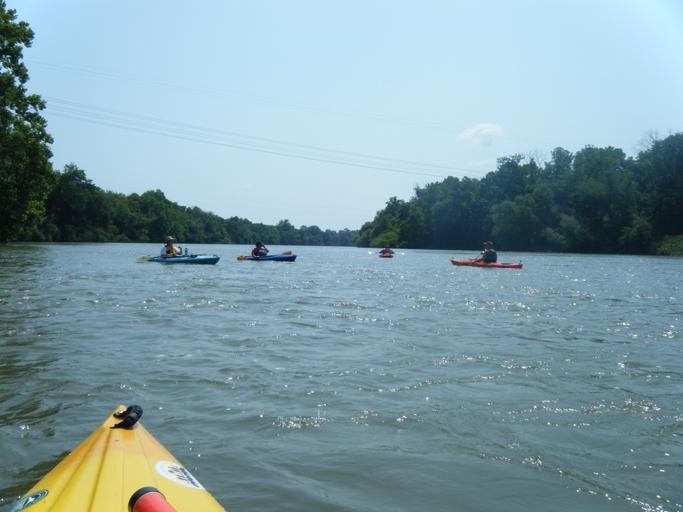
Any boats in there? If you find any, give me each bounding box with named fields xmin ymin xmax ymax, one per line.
xmin=148 ymin=255 xmax=219 ymax=265
xmin=381 ymin=253 xmax=390 ymax=257
xmin=10 ymin=404 xmax=225 ymax=511
xmin=451 ymin=258 xmax=521 ymax=269
xmin=237 ymin=254 xmax=295 ymax=261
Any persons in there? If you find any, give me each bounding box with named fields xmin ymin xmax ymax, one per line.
xmin=252 ymin=240 xmax=269 ymax=256
xmin=379 ymin=245 xmax=394 ymax=254
xmin=160 ymin=236 xmax=182 ymax=256
xmin=472 ymin=240 xmax=497 ymax=263
